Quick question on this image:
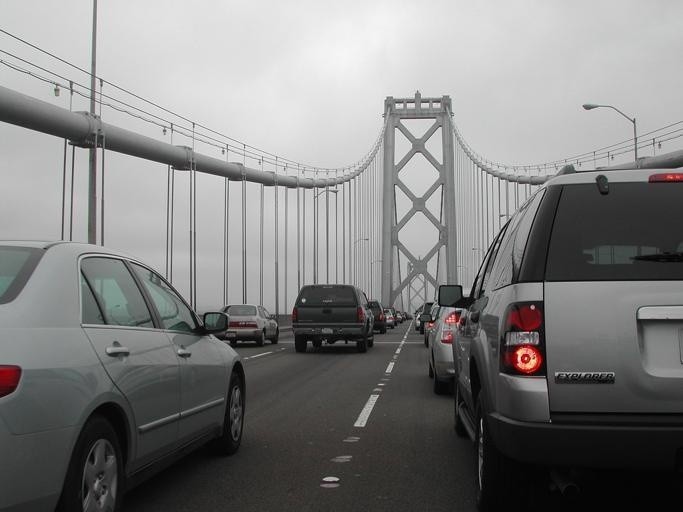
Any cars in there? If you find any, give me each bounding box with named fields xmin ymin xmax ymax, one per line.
xmin=0 ymin=241 xmax=248 ymax=512
xmin=292 ymin=285 xmax=412 ymax=351
xmin=212 ymin=304 xmax=278 ymax=347
xmin=412 ymin=294 xmax=467 ymax=394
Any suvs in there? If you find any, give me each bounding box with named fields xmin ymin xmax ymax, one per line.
xmin=436 ymin=153 xmax=683 ymax=512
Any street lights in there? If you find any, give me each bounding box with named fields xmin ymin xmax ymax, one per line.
xmin=370 ymin=259 xmax=384 ymax=300
xmin=583 ymin=104 xmax=638 ymax=160
xmin=312 ymin=188 xmax=338 ymax=284
xmin=353 ymin=238 xmax=369 ymax=285
